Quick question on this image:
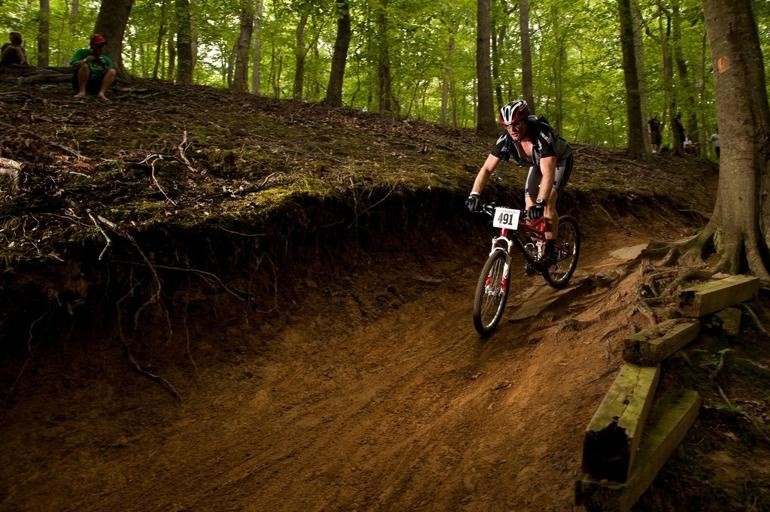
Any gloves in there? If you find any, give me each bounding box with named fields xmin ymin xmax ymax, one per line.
xmin=465 ymin=193 xmax=480 ymax=211
xmin=528 ymin=199 xmax=547 ymax=220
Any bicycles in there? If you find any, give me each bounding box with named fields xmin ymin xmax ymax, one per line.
xmin=473 ymin=203 xmax=582 ymax=337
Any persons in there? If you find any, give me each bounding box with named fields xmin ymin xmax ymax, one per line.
xmin=0 ymin=32 xmax=26 ymax=65
xmin=647 ymin=113 xmax=721 ymax=159
xmin=464 ymin=100 xmax=573 ymax=267
xmin=69 ymin=34 xmax=117 ymax=102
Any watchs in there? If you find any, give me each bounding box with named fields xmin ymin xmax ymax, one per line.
xmin=535 ymin=198 xmax=547 ymax=206
xmin=83 ymin=58 xmax=89 ymax=64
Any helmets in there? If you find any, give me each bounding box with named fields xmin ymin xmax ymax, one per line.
xmin=90 ymin=34 xmax=106 ymax=45
xmin=498 ymin=99 xmax=530 ymax=126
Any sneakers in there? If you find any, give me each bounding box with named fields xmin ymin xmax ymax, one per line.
xmin=542 ymin=243 xmax=558 ymax=263
